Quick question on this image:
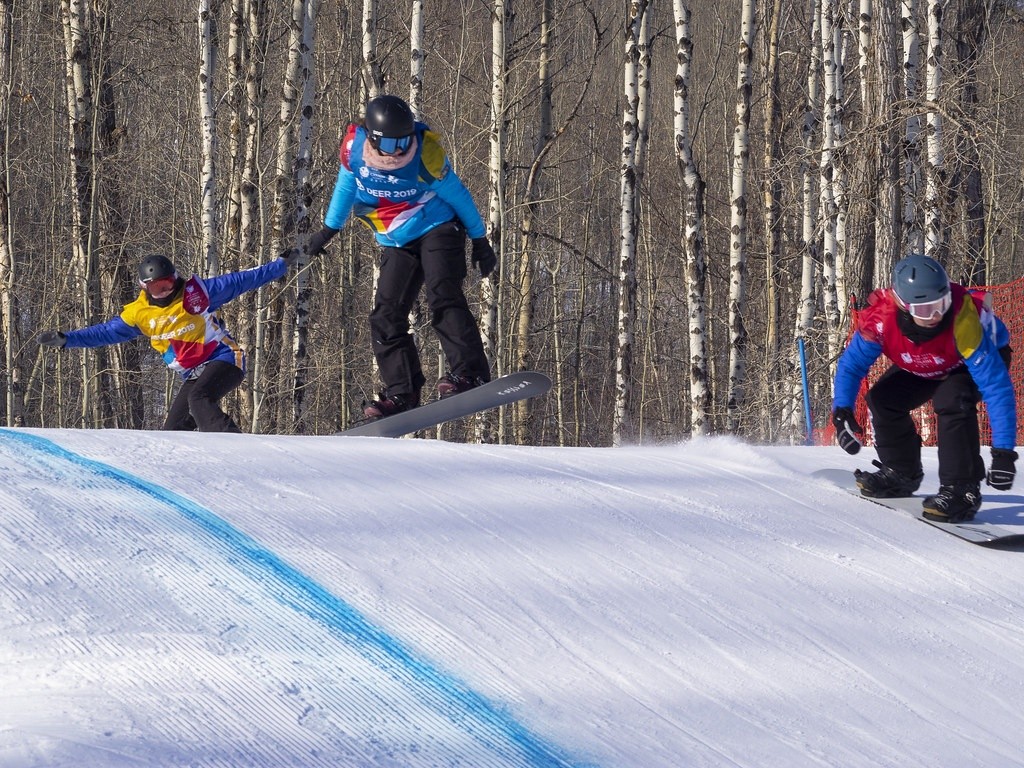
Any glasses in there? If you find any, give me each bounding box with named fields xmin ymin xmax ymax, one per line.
xmin=909 ymin=291 xmax=952 ymax=320
xmin=376 ymin=135 xmax=411 ymax=153
xmin=144 ymin=274 xmax=176 ymax=295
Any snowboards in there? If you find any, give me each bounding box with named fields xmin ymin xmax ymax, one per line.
xmin=812 ymin=467 xmax=1024 ymax=555
xmin=332 ymin=371 xmax=556 ymax=437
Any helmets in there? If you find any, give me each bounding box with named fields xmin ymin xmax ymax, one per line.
xmin=894 ymin=256 xmax=951 ymax=303
xmin=365 ymin=95 xmax=415 ymax=137
xmin=138 ymin=255 xmax=175 ymax=282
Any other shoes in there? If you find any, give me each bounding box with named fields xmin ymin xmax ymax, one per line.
xmin=436 ymin=372 xmax=479 ymax=395
xmin=854 ymin=460 xmax=924 ymax=491
xmin=923 ymin=481 xmax=982 ymax=517
xmin=363 ymin=392 xmax=409 ymax=420
xmin=37 ymin=333 xmax=66 ymax=347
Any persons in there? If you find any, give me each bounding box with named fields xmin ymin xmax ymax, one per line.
xmin=39 ymin=249 xmax=298 ymax=433
xmin=307 ymin=95 xmax=499 ymax=416
xmin=832 ymin=253 xmax=1019 ymax=522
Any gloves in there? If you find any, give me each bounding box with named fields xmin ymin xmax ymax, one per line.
xmin=304 ymin=226 xmax=339 ymax=257
xmin=988 ymin=447 xmax=1019 ymax=491
xmin=472 ymin=237 xmax=496 ymax=277
xmin=279 ymin=249 xmax=300 ymax=266
xmin=833 ymin=406 xmax=863 ymax=455
